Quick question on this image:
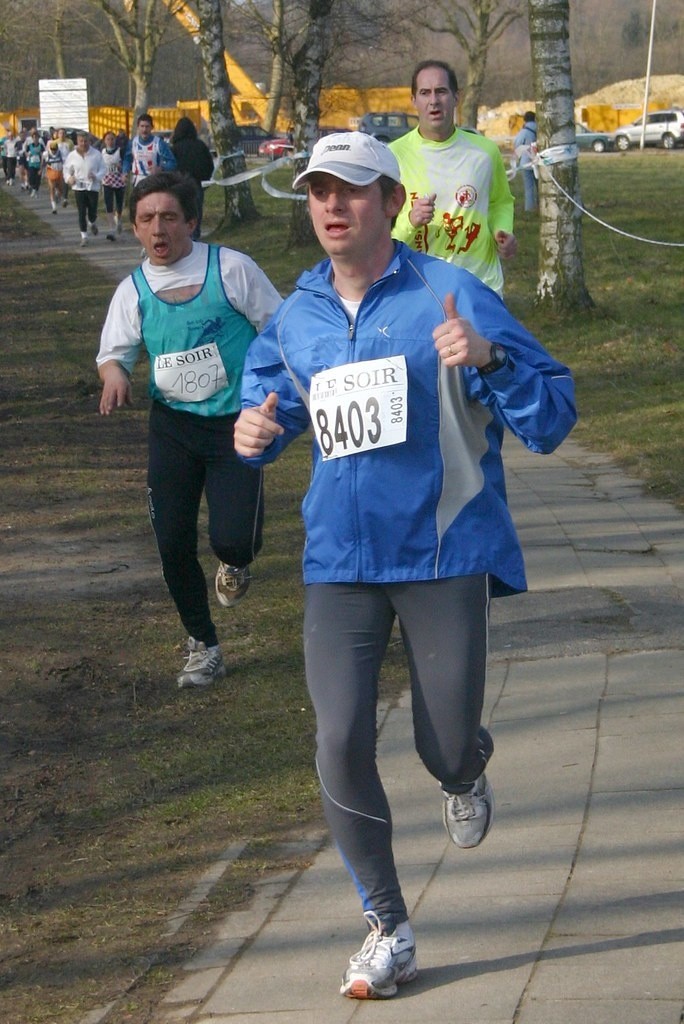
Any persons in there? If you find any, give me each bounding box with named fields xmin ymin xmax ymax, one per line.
xmin=0 ymin=116 xmax=130 ymax=248
xmin=514 ymin=111 xmax=541 ymax=215
xmin=233 ymin=131 xmax=578 ymax=999
xmin=166 ymin=117 xmax=215 ymax=241
xmin=120 ymin=113 xmax=177 ymax=188
xmin=381 ymin=61 xmax=516 ymax=301
xmin=95 ymin=172 xmax=286 ymax=689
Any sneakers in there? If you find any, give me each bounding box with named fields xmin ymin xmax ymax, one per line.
xmin=177 ymin=637 xmax=227 ymax=689
xmin=215 ymin=559 xmax=253 ymax=607
xmin=340 ymin=911 xmax=418 ymax=998
xmin=438 ymin=772 xmax=494 ymax=848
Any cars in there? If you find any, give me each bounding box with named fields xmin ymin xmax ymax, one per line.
xmin=575 ymin=122 xmax=615 ymax=153
xmin=238 ymin=124 xmax=277 ymax=139
xmin=64 ymin=128 xmax=97 ymax=145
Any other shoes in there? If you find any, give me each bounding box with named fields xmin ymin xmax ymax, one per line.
xmin=114 ymin=216 xmax=122 ymax=233
xmin=53 ymin=210 xmax=57 ymax=215
xmin=106 ymin=234 xmax=117 ymax=241
xmin=80 ymin=238 xmax=88 ymax=248
xmin=21 ymin=185 xmax=25 ymax=191
xmin=6 ymin=179 xmax=10 ymax=185
xmin=88 ymin=221 xmax=99 ymax=236
xmin=62 ymin=199 xmax=67 ymax=208
xmin=25 ymin=185 xmax=29 ymax=190
xmin=30 ymin=189 xmax=38 ymax=199
xmin=10 ymin=179 xmax=14 ymax=187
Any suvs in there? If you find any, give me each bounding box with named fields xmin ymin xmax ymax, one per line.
xmin=614 ymin=109 xmax=684 ymax=152
xmin=258 ymin=125 xmax=354 ymax=159
xmin=356 ymin=111 xmax=482 ymax=146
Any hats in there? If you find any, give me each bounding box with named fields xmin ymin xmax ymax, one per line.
xmin=50 ymin=142 xmax=58 ymax=149
xmin=291 ymin=130 xmax=400 ymax=189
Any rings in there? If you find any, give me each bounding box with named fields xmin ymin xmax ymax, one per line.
xmin=449 ymin=344 xmax=454 ymax=356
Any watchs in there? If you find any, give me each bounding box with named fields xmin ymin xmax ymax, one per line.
xmin=477 ymin=342 xmax=509 ymax=377
